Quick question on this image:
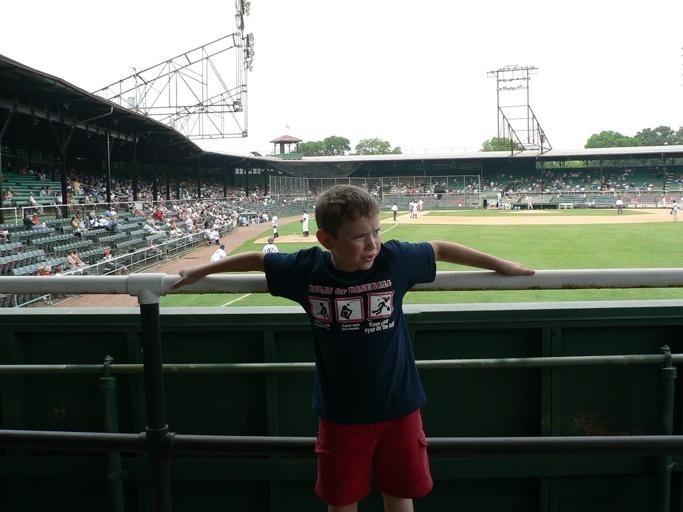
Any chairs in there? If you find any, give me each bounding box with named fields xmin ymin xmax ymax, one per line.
xmin=0 ymin=170 xmax=200 ymax=307
xmin=200 ymin=177 xmax=313 ymax=224
xmin=486 ymin=168 xmax=681 ymax=208
xmin=307 ymin=177 xmax=485 ymax=209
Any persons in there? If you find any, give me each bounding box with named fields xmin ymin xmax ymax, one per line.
xmin=171 ymin=184 xmax=534 ymax=512
